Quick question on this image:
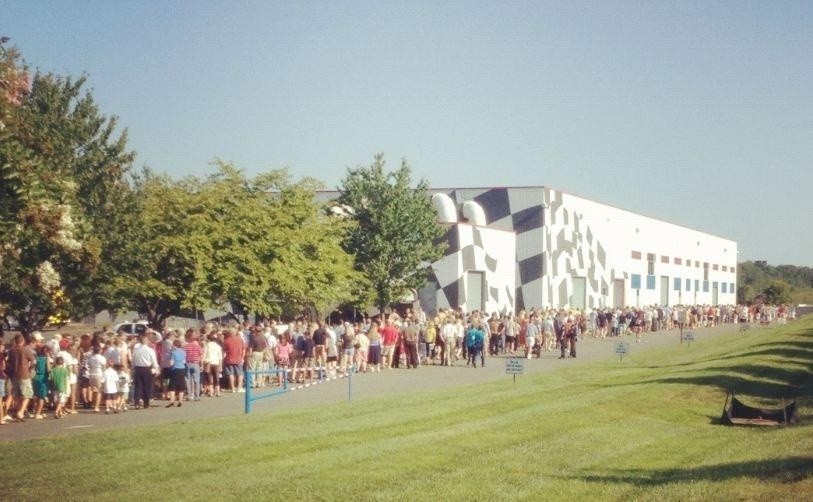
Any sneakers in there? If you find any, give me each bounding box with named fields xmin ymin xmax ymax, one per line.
xmin=53 ymin=409 xmax=78 ymax=419
xmin=1 ymin=413 xmax=48 ymax=425
xmin=83 ymin=401 xmax=149 ymax=414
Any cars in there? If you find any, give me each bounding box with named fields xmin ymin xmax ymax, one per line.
xmin=0 ymin=309 xmax=20 ymax=332
xmin=110 ymin=318 xmax=153 ymax=338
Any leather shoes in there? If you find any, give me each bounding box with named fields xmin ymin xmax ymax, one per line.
xmin=165 ymin=401 xmax=183 ymax=408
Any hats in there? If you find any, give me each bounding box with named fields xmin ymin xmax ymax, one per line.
xmin=58 ymin=338 xmax=70 ymax=348
xmin=30 ymin=329 xmax=46 ymax=340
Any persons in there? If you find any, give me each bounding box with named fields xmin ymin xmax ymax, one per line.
xmin=1 ymin=303 xmax=797 ymax=425
